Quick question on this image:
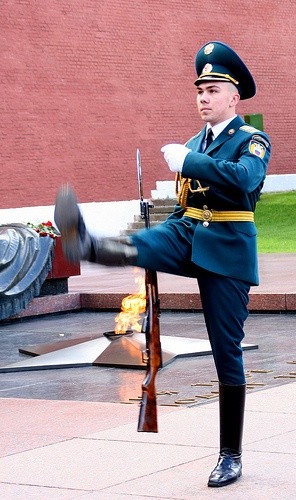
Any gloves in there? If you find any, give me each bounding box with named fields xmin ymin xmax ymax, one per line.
xmin=161 ymin=144 xmax=192 ymax=172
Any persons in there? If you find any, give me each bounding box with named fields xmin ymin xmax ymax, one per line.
xmin=54 ymin=40 xmax=271 ymax=488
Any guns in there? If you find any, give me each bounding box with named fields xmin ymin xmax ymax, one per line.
xmin=137 ymin=200 xmax=162 ymax=433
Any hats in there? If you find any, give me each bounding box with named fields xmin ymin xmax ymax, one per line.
xmin=193 ymin=41 xmax=256 ymax=100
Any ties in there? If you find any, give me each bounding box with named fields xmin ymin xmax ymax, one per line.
xmin=202 ymin=128 xmax=214 ymax=154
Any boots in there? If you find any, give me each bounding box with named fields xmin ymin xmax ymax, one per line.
xmin=54 ymin=185 xmax=138 ymax=266
xmin=208 ymin=383 xmax=246 ymax=487
